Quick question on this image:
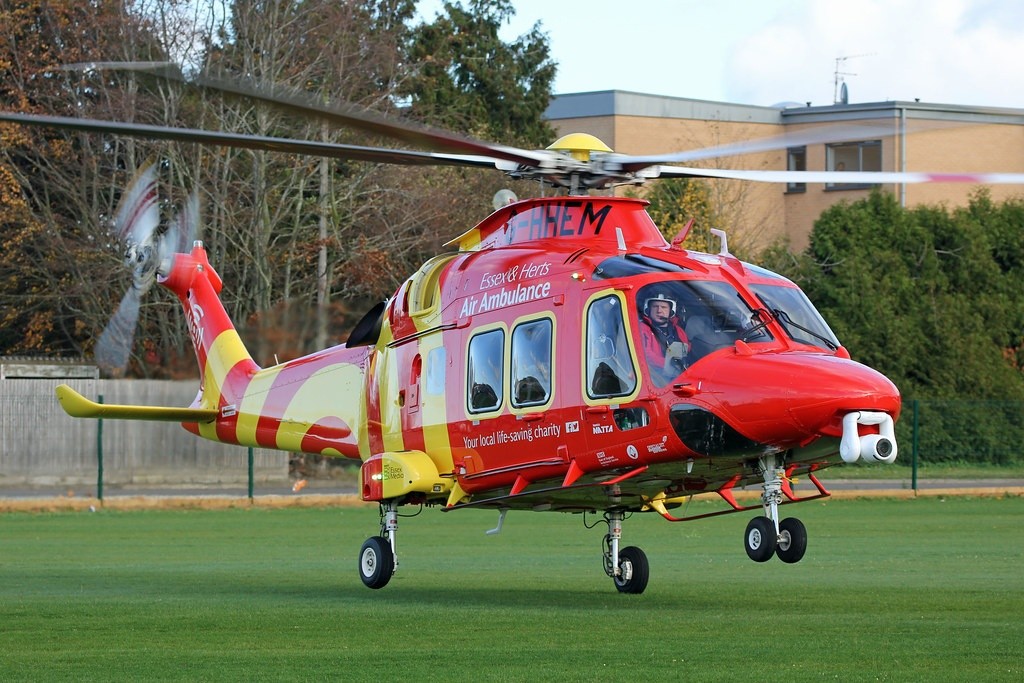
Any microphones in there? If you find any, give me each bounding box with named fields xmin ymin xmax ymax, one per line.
xmin=660 ymin=313 xmax=675 ymax=320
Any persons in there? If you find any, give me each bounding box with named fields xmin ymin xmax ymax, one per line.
xmin=637 ymin=292 xmax=689 ymax=368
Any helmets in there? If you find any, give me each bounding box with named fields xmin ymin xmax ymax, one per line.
xmin=740 ymin=304 xmax=769 ymax=333
xmin=643 ymin=288 xmax=677 ymax=319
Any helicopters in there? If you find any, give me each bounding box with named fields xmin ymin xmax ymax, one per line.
xmin=0 ymin=55 xmax=1023 ymax=591
